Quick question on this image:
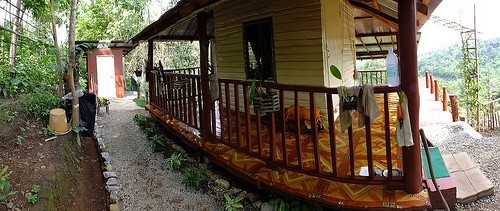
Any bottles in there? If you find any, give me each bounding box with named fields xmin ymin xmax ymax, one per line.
xmin=385 ymin=47 xmax=400 ymax=87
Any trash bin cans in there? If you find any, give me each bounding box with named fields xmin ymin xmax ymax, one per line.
xmin=64 ymin=91 xmax=97 ymax=137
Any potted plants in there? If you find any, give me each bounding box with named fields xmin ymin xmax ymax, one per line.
xmin=248 ymin=78 xmax=281 ymax=114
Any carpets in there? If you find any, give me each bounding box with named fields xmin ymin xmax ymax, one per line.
xmin=420 ymin=146 xmax=449 ymax=181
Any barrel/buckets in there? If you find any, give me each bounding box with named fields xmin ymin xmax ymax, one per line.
xmin=47 ymin=108 xmax=73 ymax=135
